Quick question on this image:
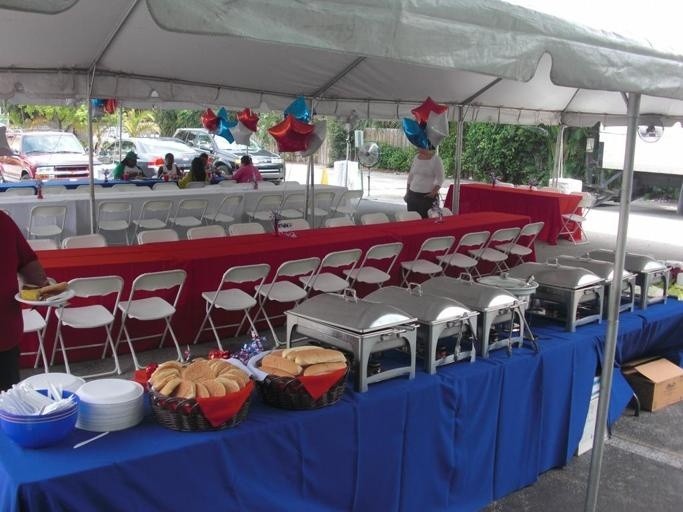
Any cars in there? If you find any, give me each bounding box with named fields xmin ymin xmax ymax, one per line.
xmin=104 ymin=138 xmax=200 ymax=179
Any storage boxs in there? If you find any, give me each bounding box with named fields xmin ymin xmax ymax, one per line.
xmin=615 ymin=349 xmax=681 ymax=413
xmin=567 ymin=374 xmax=609 ymax=458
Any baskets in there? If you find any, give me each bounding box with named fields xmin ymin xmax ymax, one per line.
xmin=248 ymin=350 xmax=351 ymax=410
xmin=148 ymin=358 xmax=252 ymax=433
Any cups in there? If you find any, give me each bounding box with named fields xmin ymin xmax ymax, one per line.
xmin=124 ymin=174 xmax=129 ymax=181
xmin=164 ymin=175 xmax=169 ymax=181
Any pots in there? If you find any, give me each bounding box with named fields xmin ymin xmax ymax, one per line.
xmin=476 ymin=274 xmax=539 ymax=297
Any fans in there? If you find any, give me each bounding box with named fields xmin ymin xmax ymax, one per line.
xmin=355 ymin=141 xmax=383 ymax=203
xmin=634 ymin=111 xmax=667 ymax=145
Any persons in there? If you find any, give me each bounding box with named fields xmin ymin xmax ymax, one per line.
xmin=178 ymin=158 xmax=209 ymax=189
xmin=0 ymin=210 xmax=49 ymax=392
xmin=158 ymin=153 xmax=182 ymax=179
xmin=200 ymin=153 xmax=222 ymax=184
xmin=113 ymin=152 xmax=143 ymax=180
xmin=232 ymin=156 xmax=262 ymax=183
xmin=404 ymin=148 xmax=445 ymax=219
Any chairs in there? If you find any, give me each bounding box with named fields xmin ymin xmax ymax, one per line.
xmin=496 ymin=181 xmax=592 ymax=198
xmin=428 ymin=207 xmax=453 ymax=218
xmin=298 ymin=222 xmax=546 ymax=304
xmin=395 ymin=211 xmax=422 ymax=223
xmin=15 ymin=256 xmax=321 ymax=380
xmin=556 ymin=197 xmax=594 ymax=245
xmin=361 ymin=212 xmax=388 ymax=226
xmin=0 ymin=165 xmax=366 ymax=252
xmin=438 ymin=188 xmax=452 ymax=207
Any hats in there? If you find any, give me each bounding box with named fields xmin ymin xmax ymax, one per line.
xmin=126 ymin=151 xmax=139 ymax=160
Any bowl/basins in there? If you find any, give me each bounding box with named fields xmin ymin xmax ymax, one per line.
xmin=3 ymin=390 xmax=78 ymax=449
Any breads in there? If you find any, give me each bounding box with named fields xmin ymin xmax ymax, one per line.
xmin=147 ymin=355 xmax=250 ymax=399
xmin=258 ymin=346 xmax=348 ymax=379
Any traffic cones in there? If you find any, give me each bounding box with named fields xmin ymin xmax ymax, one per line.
xmin=317 ymin=165 xmax=329 ymax=185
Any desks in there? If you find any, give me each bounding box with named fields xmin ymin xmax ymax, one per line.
xmin=16 ymin=232 xmax=313 ymax=367
xmin=1 ymin=291 xmax=682 ymax=511
xmin=451 ymin=183 xmax=584 ymax=245
xmin=284 ymin=213 xmax=531 ymax=293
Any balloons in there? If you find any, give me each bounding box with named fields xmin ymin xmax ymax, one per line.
xmin=104 ymin=99 xmax=118 ymax=114
xmin=201 ymin=107 xmax=259 ymax=146
xmin=269 ymin=95 xmax=327 ymax=158
xmin=91 ymin=99 xmax=106 ymax=109
xmin=403 ymin=96 xmax=449 ymax=149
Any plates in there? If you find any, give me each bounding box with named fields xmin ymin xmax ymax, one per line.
xmin=19 ymin=372 xmax=85 ymax=397
xmin=76 ymin=376 xmax=144 ymax=430
xmin=14 ymin=288 xmax=74 ymax=305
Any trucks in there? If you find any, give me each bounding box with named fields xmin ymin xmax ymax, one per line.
xmin=596 ymin=122 xmax=683 ymax=215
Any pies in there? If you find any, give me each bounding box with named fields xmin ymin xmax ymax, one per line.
xmin=21 ymin=282 xmax=68 ymax=301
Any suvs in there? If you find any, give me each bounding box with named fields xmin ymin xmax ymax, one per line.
xmin=0 ymin=126 xmax=101 ymax=187
xmin=173 ymin=128 xmax=288 ymax=186
